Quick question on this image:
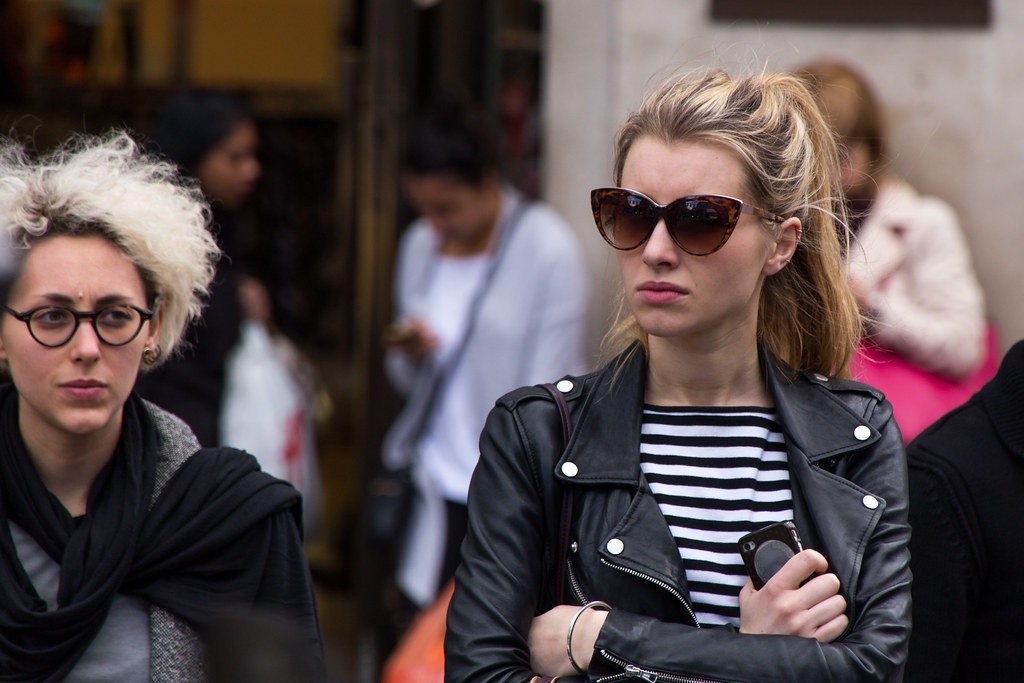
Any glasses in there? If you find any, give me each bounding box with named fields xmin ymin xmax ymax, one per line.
xmin=590 ymin=186 xmax=787 ymax=257
xmin=1 ymin=306 xmax=157 ymax=349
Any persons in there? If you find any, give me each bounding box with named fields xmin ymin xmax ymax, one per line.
xmin=905 ymin=338 xmax=1024 ymax=683
xmin=143 ymin=83 xmax=274 ymax=449
xmin=781 ymin=57 xmax=1002 ymax=446
xmin=381 ymin=111 xmax=592 ymax=683
xmin=443 ymin=15 xmax=913 ymax=683
xmin=0 ymin=112 xmax=329 ymax=683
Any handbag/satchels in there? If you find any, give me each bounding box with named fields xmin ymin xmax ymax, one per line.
xmin=341 ymin=474 xmax=410 ymax=608
xmin=219 ymin=318 xmax=319 ymax=530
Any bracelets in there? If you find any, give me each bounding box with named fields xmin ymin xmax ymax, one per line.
xmin=567 ymin=601 xmax=612 ymax=674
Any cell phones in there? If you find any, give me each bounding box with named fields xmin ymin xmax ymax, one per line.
xmin=737 ymin=522 xmax=817 ymax=592
